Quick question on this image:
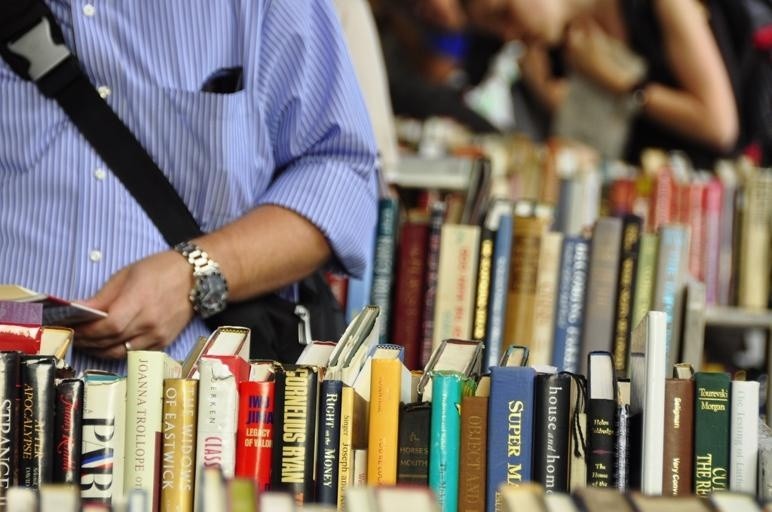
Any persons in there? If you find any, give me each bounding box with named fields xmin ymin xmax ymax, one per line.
xmin=1 ymin=0 xmax=382 ymax=381
xmin=384 ymin=1 xmax=556 ymax=147
xmin=449 ymin=2 xmax=772 ymax=175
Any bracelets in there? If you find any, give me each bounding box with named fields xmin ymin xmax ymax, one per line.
xmin=628 ymin=75 xmax=658 ymax=99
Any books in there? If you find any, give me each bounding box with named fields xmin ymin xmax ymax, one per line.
xmin=372 ymin=133 xmax=772 ymax=511
xmin=1 ymin=294 xmax=109 ymax=325
xmin=2 ymin=309 xmax=370 ymax=511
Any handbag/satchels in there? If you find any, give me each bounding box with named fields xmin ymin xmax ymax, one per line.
xmin=208 ymin=270 xmax=354 ymax=371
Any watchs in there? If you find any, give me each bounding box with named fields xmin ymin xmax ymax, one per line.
xmin=173 ymin=240 xmax=230 ymax=320
